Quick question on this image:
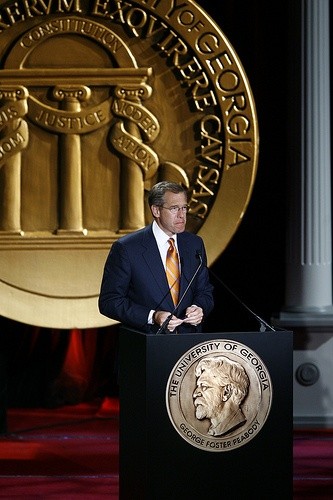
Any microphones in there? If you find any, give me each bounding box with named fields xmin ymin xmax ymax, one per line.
xmin=156 ymin=249 xmax=276 ymax=335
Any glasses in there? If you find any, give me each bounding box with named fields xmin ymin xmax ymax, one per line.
xmin=156 ymin=205 xmax=189 ymax=214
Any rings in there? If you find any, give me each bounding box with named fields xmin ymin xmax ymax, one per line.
xmin=197 ymin=319 xmax=200 ymax=322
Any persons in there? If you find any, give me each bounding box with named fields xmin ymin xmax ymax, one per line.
xmin=96 ymin=182 xmax=214 ymax=334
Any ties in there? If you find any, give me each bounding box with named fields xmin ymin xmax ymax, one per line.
xmin=166 ymin=238 xmax=180 ymax=308
